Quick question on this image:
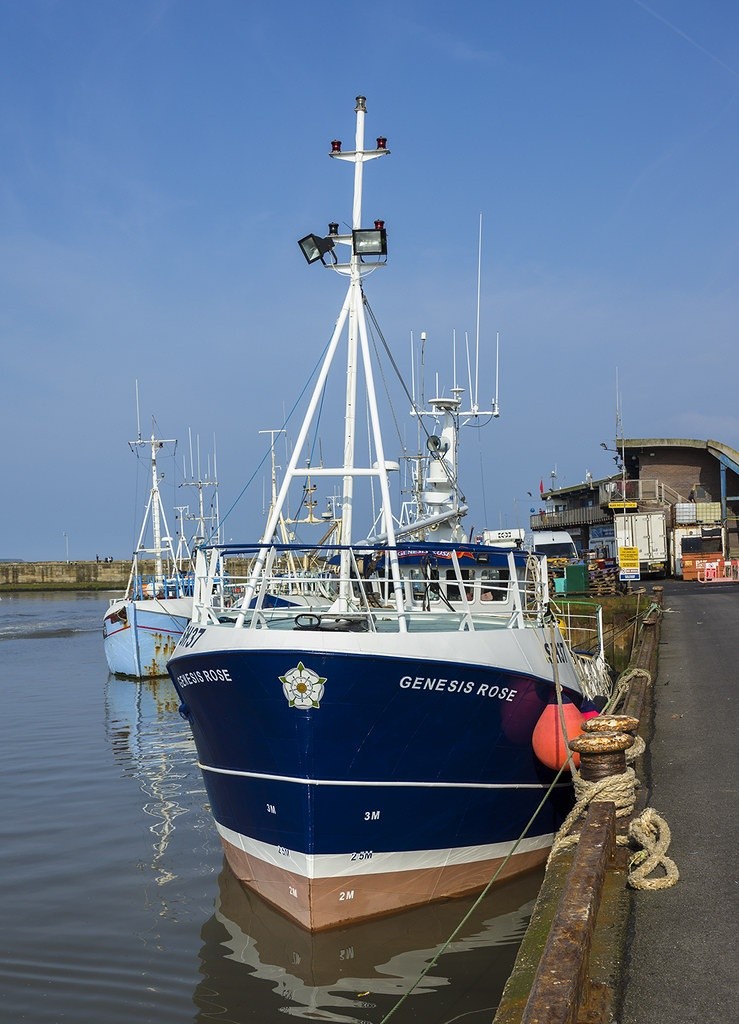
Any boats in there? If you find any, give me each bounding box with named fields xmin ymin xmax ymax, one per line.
xmin=99 ymin=88 xmax=617 ymax=939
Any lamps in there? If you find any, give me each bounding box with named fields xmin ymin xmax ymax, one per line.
xmin=352 ymin=228 xmax=387 ymax=263
xmin=613 ymin=456 xmax=623 ymax=470
xmin=600 ymin=442 xmax=618 ymax=452
xmin=298 ymin=233 xmax=337 ymax=268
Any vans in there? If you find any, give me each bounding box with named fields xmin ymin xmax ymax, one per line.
xmin=482 ymin=528 xmax=527 ymax=553
xmin=522 ymin=530 xmax=579 ymax=578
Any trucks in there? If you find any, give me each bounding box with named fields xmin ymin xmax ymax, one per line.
xmin=612 ymin=511 xmax=669 ymax=580
